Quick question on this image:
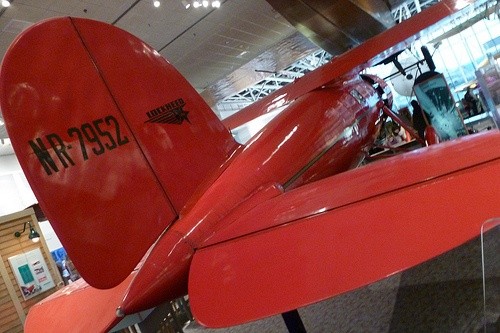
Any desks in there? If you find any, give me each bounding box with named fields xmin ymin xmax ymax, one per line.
xmin=463 ymin=112 xmax=495 ymax=135
xmin=370 ymin=139 xmax=420 ymax=157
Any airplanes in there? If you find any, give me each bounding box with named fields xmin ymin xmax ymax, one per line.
xmin=0 ymin=0 xmax=500 ymax=333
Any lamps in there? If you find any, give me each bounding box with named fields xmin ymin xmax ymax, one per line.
xmin=15 ymin=221 xmax=40 ymax=243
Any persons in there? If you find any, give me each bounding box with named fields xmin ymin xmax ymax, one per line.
xmin=464 ymin=86 xmax=479 ymax=117
xmin=411 ymin=99 xmax=432 ymax=148
